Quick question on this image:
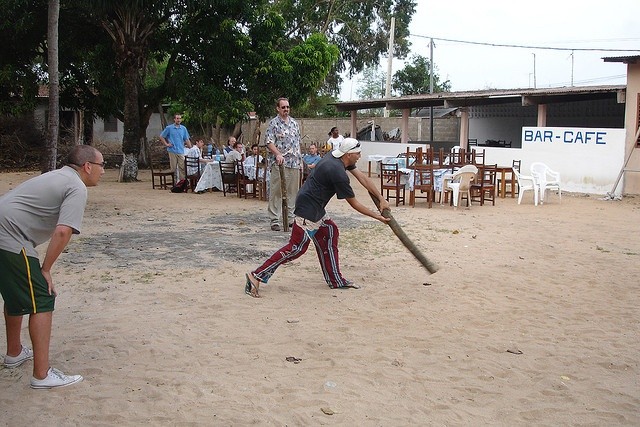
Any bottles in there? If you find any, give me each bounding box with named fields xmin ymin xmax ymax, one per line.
xmin=215 ymin=149 xmax=220 ymax=161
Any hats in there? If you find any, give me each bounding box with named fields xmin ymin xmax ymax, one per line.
xmin=331 ymin=137 xmax=362 ymax=157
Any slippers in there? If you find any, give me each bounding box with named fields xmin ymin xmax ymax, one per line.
xmin=339 ymin=280 xmax=361 ymax=289
xmin=244 ymin=273 xmax=259 ymax=297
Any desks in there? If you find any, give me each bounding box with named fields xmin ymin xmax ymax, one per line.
xmin=368 ymin=154 xmax=396 ymax=177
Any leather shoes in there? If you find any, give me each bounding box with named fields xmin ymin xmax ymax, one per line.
xmin=271 ymin=224 xmax=281 ymax=230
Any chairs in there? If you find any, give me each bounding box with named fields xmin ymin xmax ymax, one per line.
xmin=398 ymin=145 xmax=486 ymax=172
xmin=381 ymin=163 xmax=406 ymax=206
xmin=201 ymin=150 xmax=213 ymax=159
xmin=409 ymin=164 xmax=433 ymax=207
xmin=149 ymin=152 xmax=174 ymax=189
xmin=253 ymin=163 xmax=266 ymax=200
xmin=184 ymin=155 xmax=201 ymax=193
xmin=218 ymin=160 xmax=240 ymax=196
xmin=439 ymin=164 xmax=478 ymax=206
xmin=233 ymin=157 xmax=256 ymax=199
xmin=470 ymin=163 xmax=498 ymax=205
xmin=498 ymin=160 xmax=521 ymax=197
xmin=512 ymin=166 xmax=544 ymax=206
xmin=529 ymin=162 xmax=561 ymax=205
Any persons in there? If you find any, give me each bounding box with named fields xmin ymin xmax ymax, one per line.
xmin=159 ymin=112 xmax=192 ymax=186
xmin=222 ymin=141 xmax=247 ymax=193
xmin=326 ymin=126 xmax=345 ymax=151
xmin=303 ymin=144 xmax=323 ymax=170
xmin=246 ymin=136 xmax=392 ymax=299
xmin=265 ymin=96 xmax=304 ymax=231
xmin=246 ymin=143 xmax=263 ymax=166
xmin=185 ymin=137 xmax=213 ymax=193
xmin=1 ymin=145 xmax=106 ymax=390
xmin=223 ymin=136 xmax=237 ymax=159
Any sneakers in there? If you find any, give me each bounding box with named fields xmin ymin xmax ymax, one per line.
xmin=30 ymin=366 xmax=82 ymax=390
xmin=4 ymin=346 xmax=33 ymax=368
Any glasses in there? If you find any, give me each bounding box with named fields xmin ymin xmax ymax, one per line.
xmin=278 ymin=105 xmax=289 ymax=109
xmin=350 ymin=139 xmax=361 ymax=150
xmin=83 ymin=160 xmax=108 ymax=170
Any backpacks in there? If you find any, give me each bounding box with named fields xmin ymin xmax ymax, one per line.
xmin=170 ymin=178 xmax=189 ymax=193
